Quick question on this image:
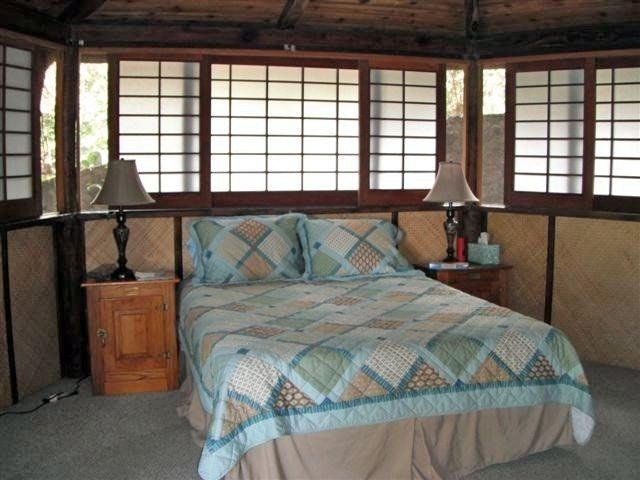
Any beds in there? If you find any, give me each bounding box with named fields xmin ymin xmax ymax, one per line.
xmin=176 ymin=271 xmax=596 ymax=480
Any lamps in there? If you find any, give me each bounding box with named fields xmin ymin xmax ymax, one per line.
xmin=422 ymin=160 xmax=480 ymax=263
xmin=90 ymin=157 xmax=156 ymax=282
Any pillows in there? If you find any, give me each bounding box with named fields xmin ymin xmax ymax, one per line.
xmin=297 ymin=218 xmax=425 ymax=282
xmin=185 ymin=212 xmax=312 ymax=287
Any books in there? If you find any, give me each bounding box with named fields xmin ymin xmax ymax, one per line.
xmin=429 ymin=261 xmax=469 ymax=271
xmin=134 ymin=270 xmax=172 ymax=280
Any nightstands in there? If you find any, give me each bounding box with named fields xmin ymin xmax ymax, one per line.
xmin=412 ymin=263 xmax=515 ymax=308
xmin=81 ymin=269 xmax=180 ymax=396
